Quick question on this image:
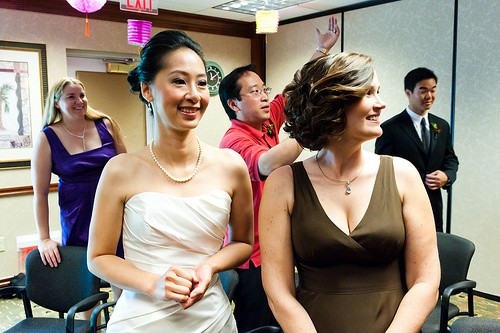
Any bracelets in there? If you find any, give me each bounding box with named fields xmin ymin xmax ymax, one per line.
xmin=442 ymin=176 xmax=450 ymax=189
xmin=316 ymin=47 xmax=328 ymax=55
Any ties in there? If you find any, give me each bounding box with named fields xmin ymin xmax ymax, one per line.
xmin=420 ymin=118 xmax=430 ymax=155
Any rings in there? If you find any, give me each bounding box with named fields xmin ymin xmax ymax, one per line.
xmin=333 ymin=30 xmax=335 ymax=33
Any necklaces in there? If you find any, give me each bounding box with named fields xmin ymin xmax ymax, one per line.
xmin=149 ymin=133 xmax=202 ymax=183
xmin=61 ymin=118 xmax=87 ymax=151
xmin=316 ymin=148 xmax=365 ymax=194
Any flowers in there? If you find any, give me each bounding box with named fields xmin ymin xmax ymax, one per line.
xmin=267 ymin=123 xmax=273 ymax=135
xmin=432 ymin=122 xmax=443 ymax=140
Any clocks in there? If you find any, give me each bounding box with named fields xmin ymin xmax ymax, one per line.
xmin=205 ymin=61 xmax=225 ymax=96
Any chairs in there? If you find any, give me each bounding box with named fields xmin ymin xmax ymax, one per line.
xmin=421 ymin=232 xmax=500 ymax=333
xmin=2 ymin=246 xmax=281 ymax=333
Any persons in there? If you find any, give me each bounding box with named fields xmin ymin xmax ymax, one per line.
xmin=258 ymin=51 xmax=441 ymax=333
xmin=30 ymin=77 xmax=127 ymax=333
xmin=217 ymin=16 xmax=341 ymax=333
xmin=375 ymin=66 xmax=460 ymax=233
xmin=86 ymin=29 xmax=255 ymax=333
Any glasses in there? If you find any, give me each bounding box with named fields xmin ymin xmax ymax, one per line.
xmin=240 ymin=87 xmax=272 ymax=98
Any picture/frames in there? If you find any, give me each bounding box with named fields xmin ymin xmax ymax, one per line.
xmin=0 ymin=41 xmax=49 ymax=170
